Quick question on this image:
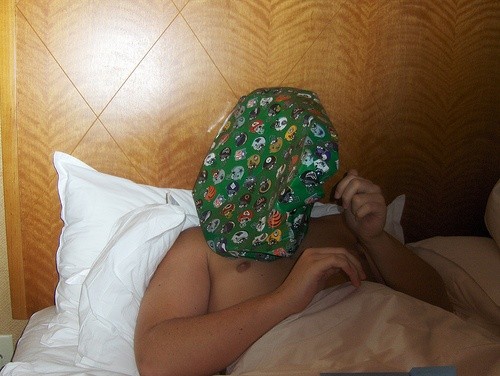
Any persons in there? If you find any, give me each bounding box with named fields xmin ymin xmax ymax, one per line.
xmin=133 ymin=87 xmax=453 ymax=376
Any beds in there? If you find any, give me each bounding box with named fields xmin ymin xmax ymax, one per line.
xmin=0 ymin=305 xmax=92 ymax=376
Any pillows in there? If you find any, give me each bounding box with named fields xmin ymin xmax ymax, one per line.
xmin=40 ymin=150 xmax=500 ymax=376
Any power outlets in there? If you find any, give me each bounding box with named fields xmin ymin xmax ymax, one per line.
xmin=0 ymin=336 xmax=13 ymax=369
